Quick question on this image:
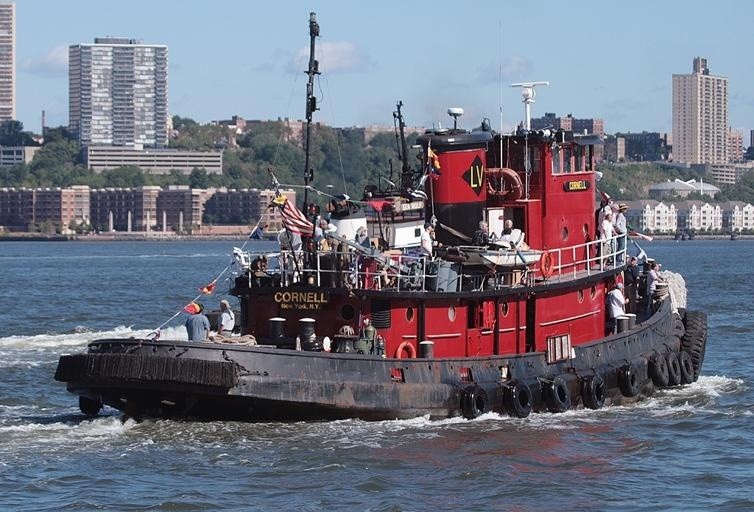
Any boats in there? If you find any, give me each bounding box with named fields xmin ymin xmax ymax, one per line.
xmin=53 ymin=10 xmax=710 ymax=422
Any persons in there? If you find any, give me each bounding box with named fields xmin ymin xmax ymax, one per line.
xmin=358 ymin=314 xmax=377 ymax=357
xmin=597 ymin=212 xmax=618 ymax=272
xmin=594 ymin=199 xmax=630 ymax=265
xmin=373 ymin=333 xmax=388 ymax=357
xmin=418 ymin=221 xmax=433 ymax=291
xmin=335 ymin=324 xmax=355 ymax=356
xmin=471 ymin=221 xmax=494 ymax=291
xmin=244 ymin=203 xmax=395 ymax=291
xmin=623 ymin=255 xmax=663 ymax=321
xmin=500 ymin=219 xmax=514 ymax=237
xmin=183 ymin=302 xmax=210 ymax=342
xmin=217 ymin=299 xmax=235 ymax=338
xmin=429 ymin=223 xmax=443 ymax=258
xmin=604 ymin=283 xmax=629 ymax=337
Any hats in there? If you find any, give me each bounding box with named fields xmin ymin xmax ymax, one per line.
xmin=618 ymin=203 xmax=629 ymax=211
xmin=424 ymin=223 xmax=432 ymax=228
xmin=616 ymin=284 xmax=622 ymax=289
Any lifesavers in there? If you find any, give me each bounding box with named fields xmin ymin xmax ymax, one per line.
xmin=396 ymin=339 xmax=416 ymax=360
xmin=540 ymin=252 xmax=555 ymax=278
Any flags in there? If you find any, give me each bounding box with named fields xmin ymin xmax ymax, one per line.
xmin=270 ymin=177 xmax=316 ymax=239
xmin=427 ymin=147 xmax=441 ymax=169
xmin=424 ymin=164 xmax=441 ymax=180
